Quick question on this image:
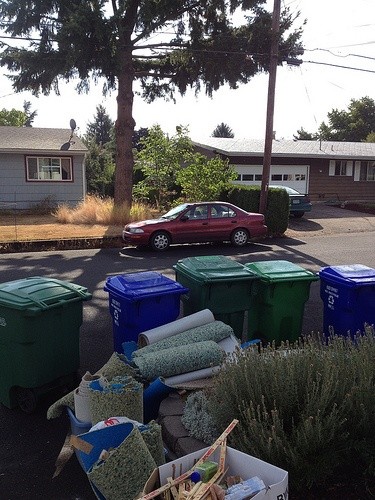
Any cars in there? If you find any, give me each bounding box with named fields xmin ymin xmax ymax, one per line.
xmin=268 ymin=184 xmax=312 ymax=217
xmin=122 ymin=200 xmax=266 ymax=251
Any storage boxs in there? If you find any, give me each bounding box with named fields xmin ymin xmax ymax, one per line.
xmin=142 ymin=446 xmax=288 ymax=500
xmin=64 ymin=408 xmax=91 ymax=436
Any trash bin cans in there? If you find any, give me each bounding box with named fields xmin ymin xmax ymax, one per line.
xmin=104 ymin=271 xmax=189 ymax=354
xmin=0 ymin=278 xmax=92 ymax=413
xmin=244 ymin=261 xmax=318 ymax=350
xmin=317 ymin=263 xmax=375 ymax=343
xmin=173 ymin=255 xmax=259 ymax=345
